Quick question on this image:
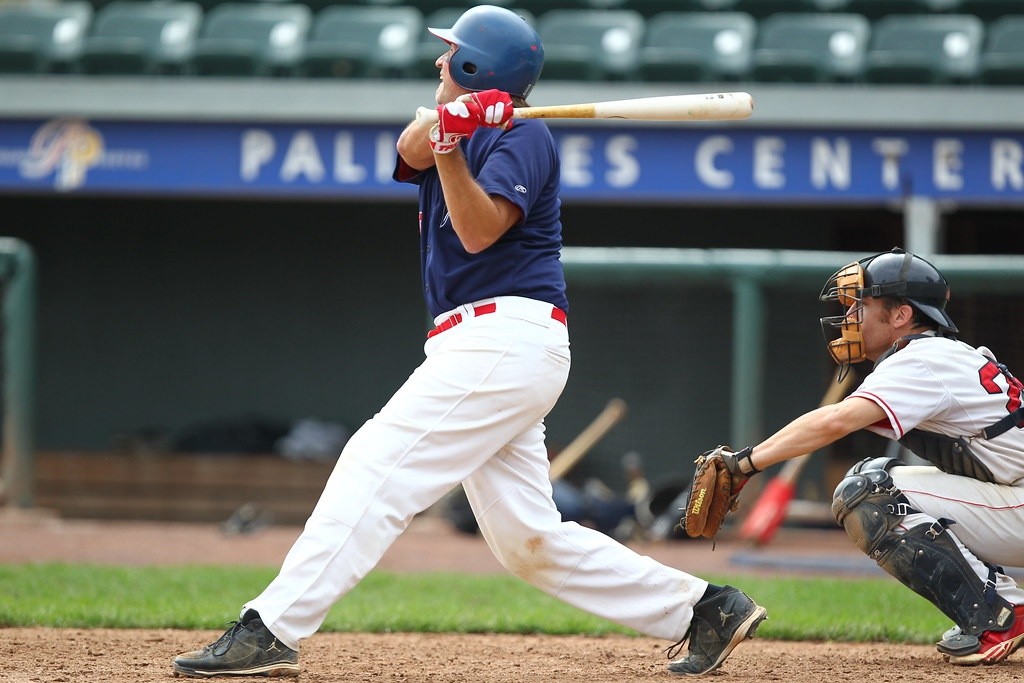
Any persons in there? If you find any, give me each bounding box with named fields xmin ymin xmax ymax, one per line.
xmin=686 ymin=246 xmax=1024 ymax=666
xmin=173 ymin=5 xmax=764 ymax=676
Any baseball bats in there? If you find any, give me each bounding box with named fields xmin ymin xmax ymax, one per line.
xmin=415 ymin=90 xmax=755 ymax=128
xmin=737 ymin=357 xmax=857 ymax=550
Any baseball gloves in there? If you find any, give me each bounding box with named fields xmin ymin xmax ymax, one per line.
xmin=683 ymin=444 xmax=755 ymax=541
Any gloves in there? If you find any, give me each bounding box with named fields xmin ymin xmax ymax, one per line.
xmin=427 ymin=88 xmax=516 ymax=154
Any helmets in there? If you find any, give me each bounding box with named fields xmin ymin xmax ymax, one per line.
xmin=854 ymin=246 xmax=960 ymax=334
xmin=428 ymin=5 xmax=546 ymax=100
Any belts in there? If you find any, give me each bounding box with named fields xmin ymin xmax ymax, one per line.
xmin=427 ymin=303 xmax=566 ymax=337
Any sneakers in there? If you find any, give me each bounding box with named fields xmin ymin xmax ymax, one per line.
xmin=174 ymin=618 xmax=301 ymax=677
xmin=667 ymin=584 xmax=768 ymax=676
xmin=943 ymin=604 xmax=1024 ymax=665
